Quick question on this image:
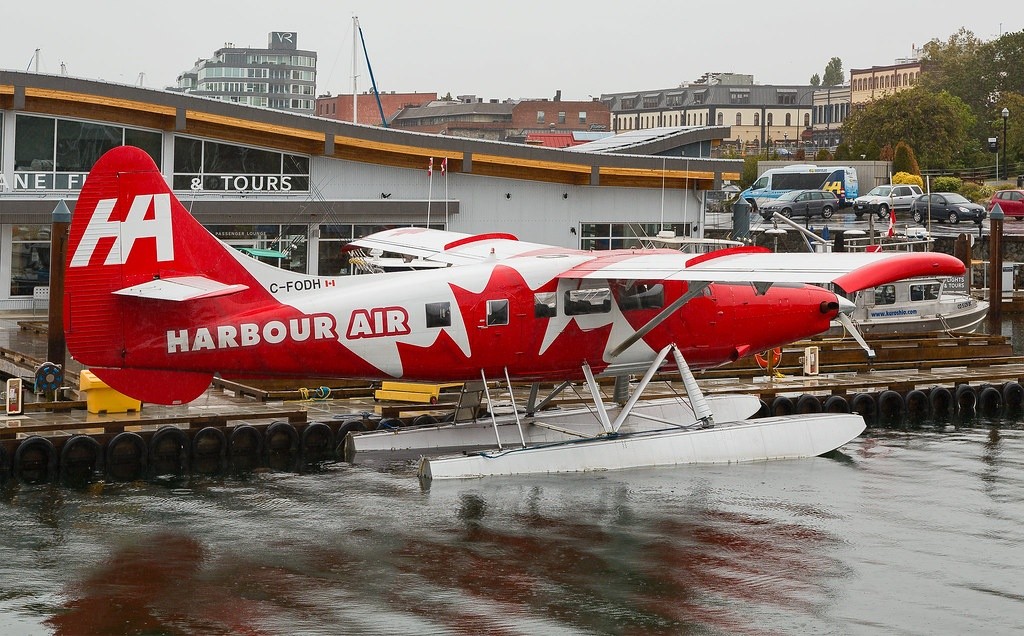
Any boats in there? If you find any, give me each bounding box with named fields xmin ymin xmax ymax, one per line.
xmin=750 ymin=208 xmax=991 ymax=343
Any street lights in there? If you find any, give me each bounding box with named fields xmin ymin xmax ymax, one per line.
xmin=796 ymin=90 xmax=819 ymax=149
xmin=1001 ymin=107 xmax=1010 ymax=182
xmin=838 ymin=98 xmax=855 ymax=114
xmin=783 ymin=131 xmax=789 ymax=148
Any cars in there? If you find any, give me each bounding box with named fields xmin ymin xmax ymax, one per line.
xmin=988 ymin=188 xmax=1024 ymax=223
xmin=909 ymin=190 xmax=988 ymax=225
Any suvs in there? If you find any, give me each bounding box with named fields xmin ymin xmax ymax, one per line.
xmin=852 ymin=183 xmax=923 ymax=219
xmin=759 ymin=189 xmax=840 ymax=222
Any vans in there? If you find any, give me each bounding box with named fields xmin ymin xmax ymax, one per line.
xmin=739 ymin=163 xmax=860 ymax=212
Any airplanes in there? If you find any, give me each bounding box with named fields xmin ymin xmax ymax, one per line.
xmin=60 ymin=143 xmax=969 ymax=484
